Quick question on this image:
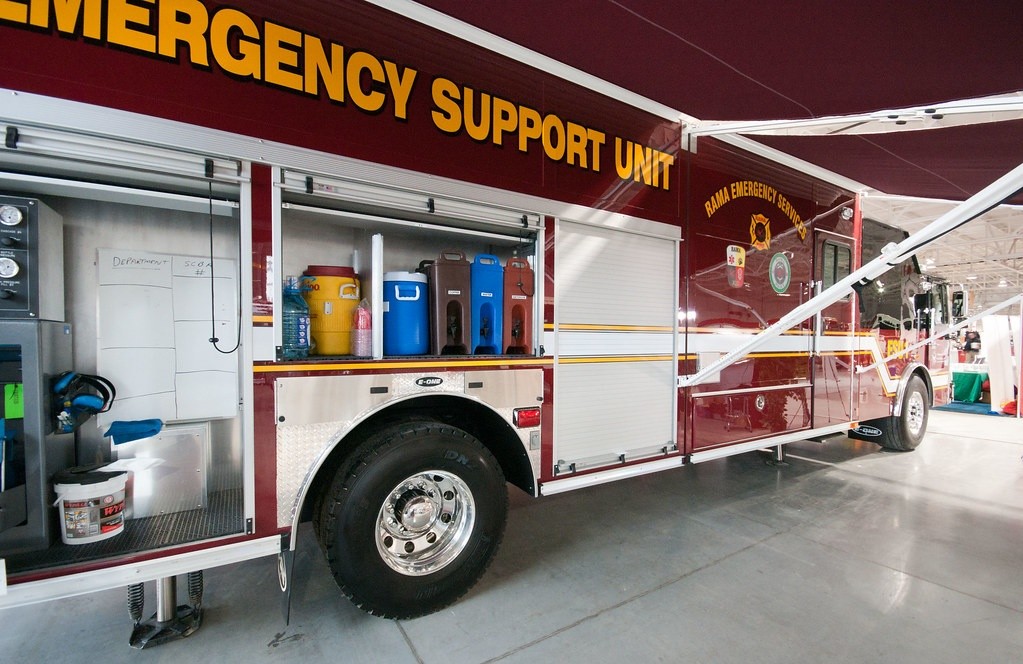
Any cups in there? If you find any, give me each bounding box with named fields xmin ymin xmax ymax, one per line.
xmin=354 ymin=308 xmax=372 ymax=356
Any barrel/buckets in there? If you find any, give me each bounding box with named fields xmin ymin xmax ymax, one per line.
xmin=298 ymin=266 xmax=360 ymax=355
xmin=383 ymin=271 xmax=429 ymax=355
xmin=53 ymin=468 xmax=128 ymax=545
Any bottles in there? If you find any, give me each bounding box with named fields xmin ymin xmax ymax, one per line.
xmin=282 ymin=276 xmax=316 ymax=359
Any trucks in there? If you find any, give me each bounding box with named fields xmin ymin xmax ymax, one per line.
xmin=0 ymin=0 xmax=966 ymax=649
